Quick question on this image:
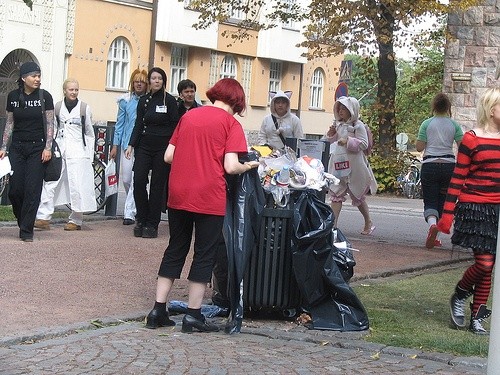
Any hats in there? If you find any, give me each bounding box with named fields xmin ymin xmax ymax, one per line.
xmin=15 ymin=62 xmax=40 ymax=83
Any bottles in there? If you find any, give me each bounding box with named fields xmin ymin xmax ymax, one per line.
xmin=279 ymin=164 xmax=290 ymax=184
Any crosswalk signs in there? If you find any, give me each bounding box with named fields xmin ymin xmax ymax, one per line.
xmin=338 ymin=60 xmax=353 ymax=84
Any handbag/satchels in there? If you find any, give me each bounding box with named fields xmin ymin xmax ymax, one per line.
xmin=42 ymin=139 xmax=62 ymax=182
xmin=103 ymin=158 xmax=118 ymax=198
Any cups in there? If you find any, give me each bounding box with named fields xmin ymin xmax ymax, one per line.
xmin=333 ymin=241 xmax=347 ymax=248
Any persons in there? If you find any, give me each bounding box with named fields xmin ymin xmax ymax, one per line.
xmin=110 ymin=68 xmax=150 ymax=224
xmin=34 ymin=78 xmax=97 ymax=231
xmin=438 ymin=87 xmax=500 ymax=335
xmin=259 ymin=90 xmax=305 ymax=149
xmin=125 ymin=67 xmax=180 ymax=238
xmin=176 ymin=79 xmax=202 ymax=113
xmin=147 ymin=78 xmax=259 ymax=332
xmin=416 ymin=94 xmax=464 ymax=248
xmin=0 ymin=62 xmax=54 ymax=242
xmin=320 ymin=96 xmax=379 ymax=235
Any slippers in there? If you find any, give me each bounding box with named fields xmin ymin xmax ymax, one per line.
xmin=360 ymin=225 xmax=376 ymax=236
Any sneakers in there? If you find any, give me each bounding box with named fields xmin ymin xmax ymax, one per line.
xmin=466 ymin=300 xmax=492 ymax=335
xmin=449 ymin=284 xmax=473 ymax=330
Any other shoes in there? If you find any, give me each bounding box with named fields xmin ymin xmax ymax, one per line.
xmin=123 ymin=218 xmax=135 ymax=225
xmin=19 ymin=231 xmax=33 ymax=242
xmin=425 ymin=224 xmax=442 ymax=249
xmin=33 ymin=219 xmax=50 ymax=231
xmin=63 ymin=222 xmax=81 ymax=231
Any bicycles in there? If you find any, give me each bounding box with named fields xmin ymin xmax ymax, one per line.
xmin=384 ymin=152 xmax=423 ymax=199
xmin=0 ymin=150 xmax=111 ymax=216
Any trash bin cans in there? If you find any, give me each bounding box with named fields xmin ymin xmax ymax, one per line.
xmin=232 ymin=182 xmax=327 ymax=318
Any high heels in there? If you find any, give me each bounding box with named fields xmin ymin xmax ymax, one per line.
xmin=181 ymin=315 xmax=219 ymax=333
xmin=133 ymin=223 xmax=158 ymax=238
xmin=145 ymin=309 xmax=171 ymax=329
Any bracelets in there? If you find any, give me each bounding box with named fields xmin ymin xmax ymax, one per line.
xmin=246 ymin=163 xmax=252 ymax=169
